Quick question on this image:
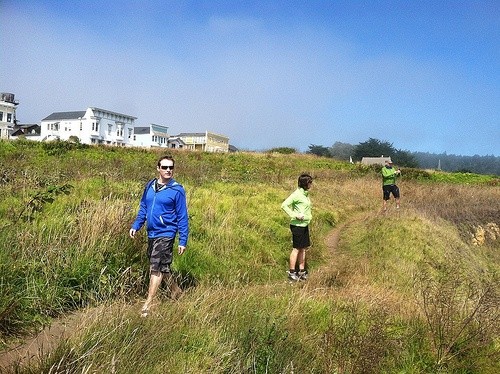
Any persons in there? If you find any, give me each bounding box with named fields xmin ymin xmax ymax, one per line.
xmin=283 ymin=175 xmax=313 ymax=284
xmin=379 ymin=159 xmax=402 ymax=218
xmin=130 ymin=157 xmax=190 ymax=319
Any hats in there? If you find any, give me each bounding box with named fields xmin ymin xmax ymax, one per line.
xmin=385 ymin=159 xmax=393 ymax=164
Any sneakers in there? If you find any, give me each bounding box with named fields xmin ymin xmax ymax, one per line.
xmin=288 ymin=270 xmax=302 ymax=281
xmin=298 ymin=271 xmax=308 ymax=280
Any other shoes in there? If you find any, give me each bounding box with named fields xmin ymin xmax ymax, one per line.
xmin=396 ymin=204 xmax=400 ymax=209
xmin=171 ymin=288 xmax=182 ymax=302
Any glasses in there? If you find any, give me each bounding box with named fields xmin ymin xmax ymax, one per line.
xmin=161 ymin=166 xmax=174 ymax=170
